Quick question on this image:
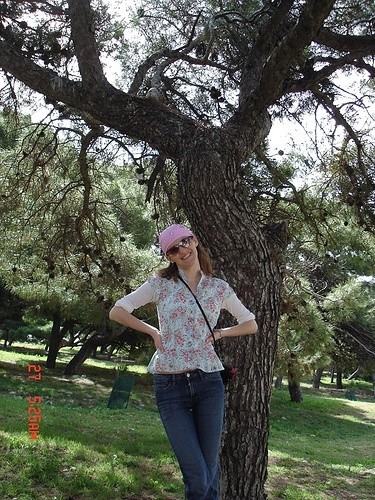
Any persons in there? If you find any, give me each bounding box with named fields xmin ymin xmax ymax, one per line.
xmin=108 ymin=224 xmax=259 ymax=500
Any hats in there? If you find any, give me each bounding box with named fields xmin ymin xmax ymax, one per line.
xmin=159 ymin=224 xmax=193 ymax=255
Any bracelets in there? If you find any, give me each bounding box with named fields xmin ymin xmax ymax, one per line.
xmin=219 ymin=329 xmax=222 ymax=338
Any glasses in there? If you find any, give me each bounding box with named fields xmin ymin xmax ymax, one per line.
xmin=167 ymin=236 xmax=193 ymax=256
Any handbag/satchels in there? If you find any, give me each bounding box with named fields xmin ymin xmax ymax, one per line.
xmin=219 ymin=361 xmax=237 ymax=386
